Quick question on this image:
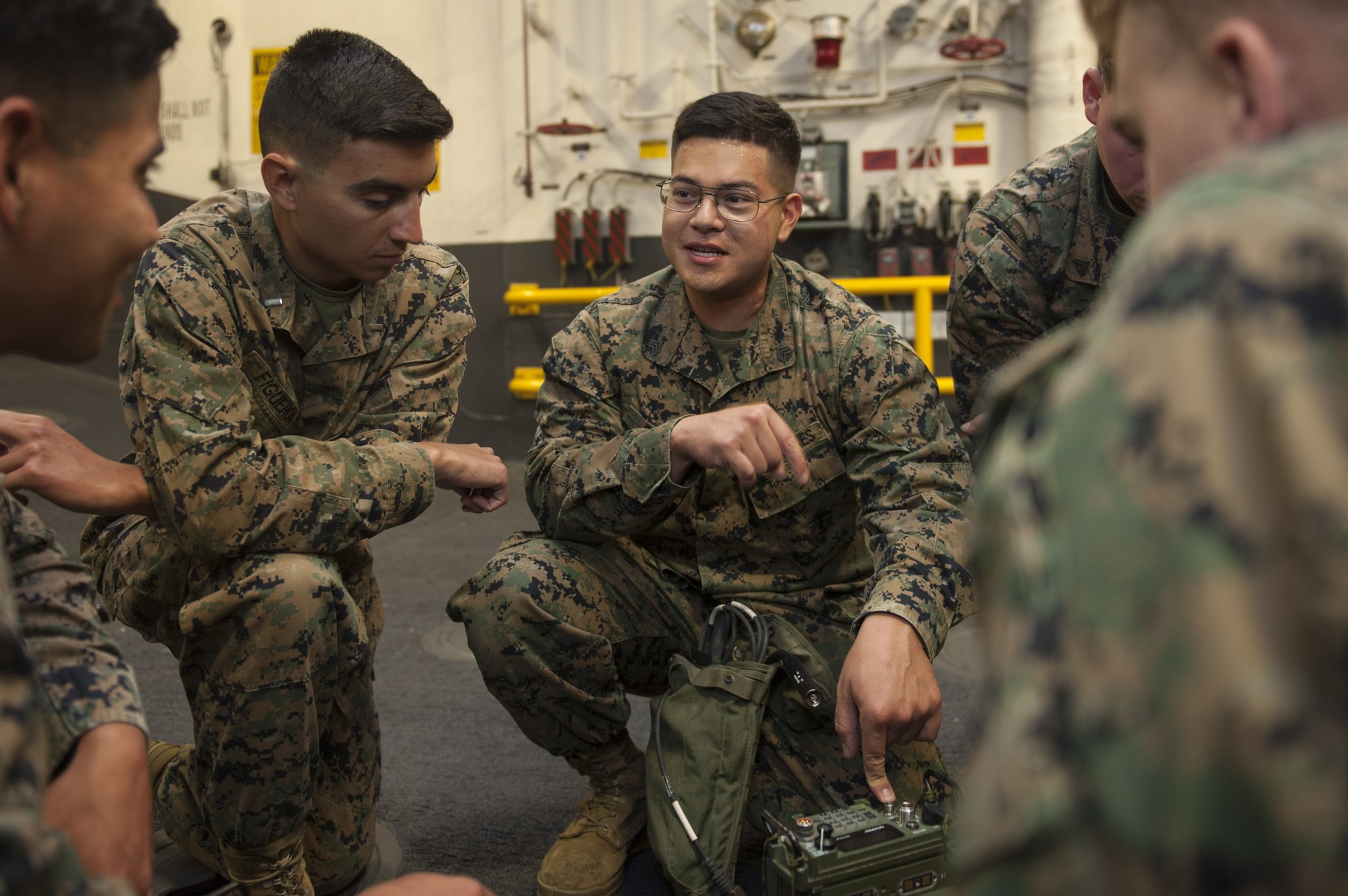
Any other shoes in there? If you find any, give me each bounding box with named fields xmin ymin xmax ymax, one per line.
xmin=239 ymin=860 xmax=314 ymax=896
xmin=150 ymin=739 xmax=202 ymax=875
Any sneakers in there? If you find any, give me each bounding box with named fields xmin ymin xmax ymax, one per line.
xmin=535 ymin=754 xmax=641 ymax=896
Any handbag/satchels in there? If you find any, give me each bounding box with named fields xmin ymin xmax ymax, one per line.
xmin=643 ymin=601 xmax=777 ymax=890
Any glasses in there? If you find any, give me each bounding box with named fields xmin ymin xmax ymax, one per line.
xmin=658 ymin=177 xmax=786 ymax=223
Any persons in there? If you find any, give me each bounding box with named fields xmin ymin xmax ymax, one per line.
xmin=0 ymin=31 xmax=510 ymax=896
xmin=939 ymin=0 xmax=1347 ymax=896
xmin=2 ymin=1 xmax=496 ymax=895
xmin=949 ymin=0 xmax=1155 ymax=469
xmin=447 ymin=90 xmax=976 ymax=896
